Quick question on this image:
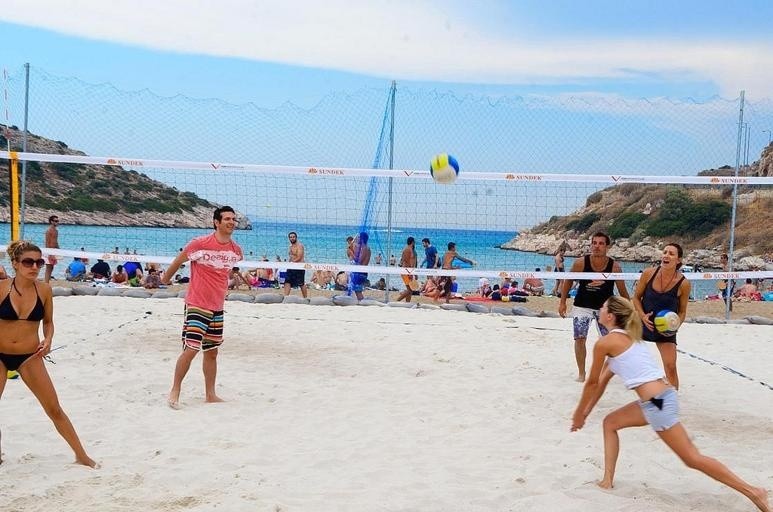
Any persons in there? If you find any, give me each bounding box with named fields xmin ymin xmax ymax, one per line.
xmin=349 ymin=232 xmax=371 ymax=300
xmin=80 ymin=247 xmax=89 ymax=264
xmin=719 ymin=253 xmax=738 ymax=313
xmin=733 ymin=278 xmax=761 ymax=303
xmin=389 ymin=255 xmax=396 ymax=265
xmin=633 ymin=270 xmax=644 ymax=292
xmin=398 ymin=241 xmax=418 ymax=268
xmin=633 ymin=242 xmax=692 ymax=392
xmin=345 ymin=237 xmax=356 ymax=261
xmin=180 ymin=264 xmax=190 ymax=274
xmin=179 ymin=248 xmax=183 ymax=253
xmin=552 ymin=247 xmax=566 ymax=297
xmin=374 ymin=253 xmax=383 ymax=265
xmin=174 ymin=274 xmax=191 ymax=284
xmin=449 ymin=276 xmax=458 ymax=299
xmin=89 ymin=259 xmax=111 ymax=284
xmin=372 ymin=278 xmax=386 ymax=290
xmin=111 ymin=264 xmax=128 ymax=288
xmin=761 ymin=282 xmax=773 ymax=302
xmin=132 ymin=249 xmax=137 ymax=255
xmin=160 ymin=205 xmax=244 ymax=411
xmin=282 ymin=232 xmax=307 ymax=298
xmin=42 ymin=215 xmax=59 ymax=284
xmin=228 ymin=266 xmax=251 ymax=291
xmin=144 ymin=262 xmax=163 ymax=276
xmin=123 ymin=248 xmax=131 ymax=255
xmin=751 ymin=266 xmax=767 ymax=289
xmin=246 ymin=251 xmax=287 ymax=288
xmin=420 ymin=237 xmax=442 ymax=295
xmin=0 ymin=240 xmax=98 ymax=469
xmin=310 ymin=270 xmax=326 ymax=290
xmin=334 ymin=271 xmax=349 ymax=291
xmin=558 ymin=231 xmax=632 ymax=382
xmin=396 ymin=236 xmax=418 ymax=304
xmin=112 ymin=246 xmax=119 ymax=254
xmin=475 ymin=265 xmax=553 ymax=303
xmin=718 ymin=279 xmax=738 ymax=299
xmin=135 ymin=268 xmax=160 ymax=289
xmin=568 ymin=295 xmax=772 ymax=511
xmin=320 ymin=271 xmax=336 ymax=289
xmin=433 ymin=241 xmax=474 ymax=304
xmin=64 ymin=257 xmax=86 ymax=282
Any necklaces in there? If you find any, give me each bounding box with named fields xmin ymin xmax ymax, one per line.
xmin=591 ymin=256 xmax=608 ymax=270
xmin=661 ymin=268 xmax=676 ymax=293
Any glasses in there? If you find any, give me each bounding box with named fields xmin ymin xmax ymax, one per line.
xmin=21 ymin=258 xmax=46 ymax=268
xmin=53 ymin=220 xmax=59 ymax=222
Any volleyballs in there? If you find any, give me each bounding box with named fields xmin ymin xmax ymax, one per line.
xmin=430 ymin=154 xmax=460 ymax=184
xmin=654 ymin=310 xmax=680 ymax=337
